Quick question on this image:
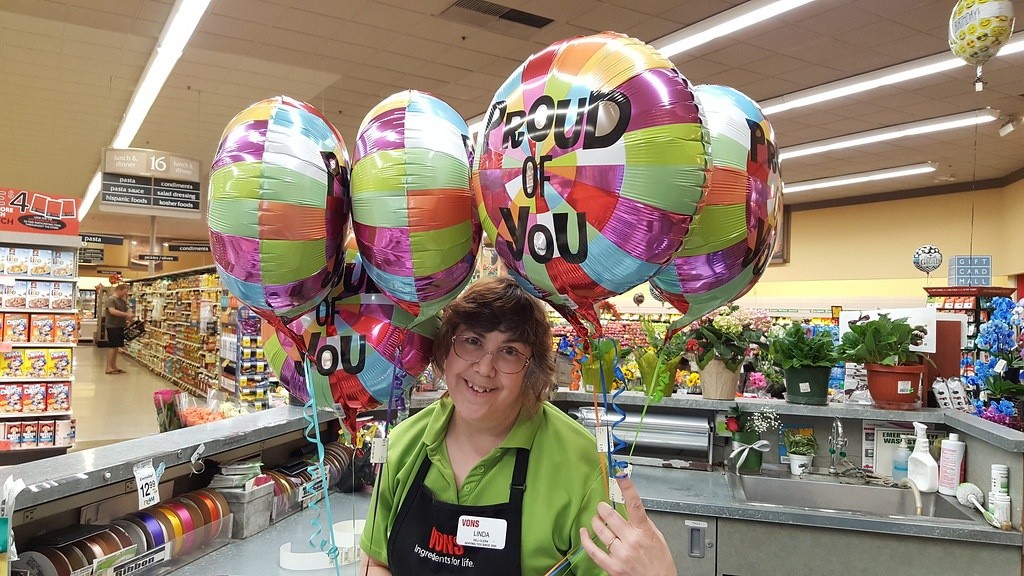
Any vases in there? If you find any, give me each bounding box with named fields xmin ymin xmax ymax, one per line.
xmin=732 ymin=433 xmax=763 ymax=476
xmin=577 ymin=347 xmax=622 ymax=393
xmin=697 ymin=352 xmax=745 ymax=400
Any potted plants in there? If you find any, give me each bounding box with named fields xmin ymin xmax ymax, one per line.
xmin=786 ymin=429 xmax=817 ymax=477
xmin=749 ymin=319 xmax=839 ymax=405
xmin=627 ymin=311 xmax=684 ymax=396
xmin=836 ymin=312 xmax=937 ymax=410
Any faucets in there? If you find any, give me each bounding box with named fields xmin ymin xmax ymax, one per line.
xmin=898 ymin=478 xmax=923 ymax=515
xmin=827 ymin=416 xmax=848 ymax=474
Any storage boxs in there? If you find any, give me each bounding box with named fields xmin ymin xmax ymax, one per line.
xmin=272 ymin=486 xmax=301 ymax=525
xmin=90 ymin=514 xmax=234 ymax=576
xmin=218 ymin=481 xmax=276 ymax=538
xmin=0 ymin=245 xmax=76 ymax=418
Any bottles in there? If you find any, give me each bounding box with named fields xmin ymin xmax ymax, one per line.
xmin=988 ymin=465 xmax=1011 ymax=527
xmin=937 ymin=432 xmax=967 ymax=497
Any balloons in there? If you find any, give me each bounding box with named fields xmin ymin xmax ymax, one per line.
xmin=913 ymin=245 xmax=943 ymax=273
xmin=947 ymin=0 xmax=1016 ymax=65
xmin=209 ymin=30 xmax=784 ymax=446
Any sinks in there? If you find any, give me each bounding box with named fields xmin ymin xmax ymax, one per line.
xmin=726 ymin=471 xmax=975 ymax=526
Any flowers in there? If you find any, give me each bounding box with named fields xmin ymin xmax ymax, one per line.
xmin=959 ymin=295 xmax=1024 ymax=432
xmin=152 ymin=386 xmax=261 ymax=434
xmin=596 ymin=297 xmax=780 ymax=394
xmin=723 ymin=403 xmax=784 ymax=434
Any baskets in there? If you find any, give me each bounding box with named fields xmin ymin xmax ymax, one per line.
xmin=121 ymin=321 xmax=146 ymax=341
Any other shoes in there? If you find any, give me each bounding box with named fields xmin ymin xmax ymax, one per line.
xmin=115 ymin=369 xmax=125 ymax=373
xmin=105 ymin=370 xmax=119 ymax=373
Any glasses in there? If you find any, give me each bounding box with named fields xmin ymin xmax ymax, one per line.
xmin=451 ymin=334 xmax=537 ymax=375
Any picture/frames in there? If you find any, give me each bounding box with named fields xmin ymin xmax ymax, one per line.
xmin=768 ymin=205 xmax=793 ymax=266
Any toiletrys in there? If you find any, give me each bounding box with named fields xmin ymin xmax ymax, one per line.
xmin=890 ymin=434 xmax=916 ymax=484
xmin=907 ymin=421 xmax=939 ymax=492
xmin=938 ymin=433 xmax=966 ymax=497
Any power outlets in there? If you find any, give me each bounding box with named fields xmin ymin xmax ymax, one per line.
xmin=23 ymin=508 xmax=38 ymax=523
xmin=259 ymin=440 xmax=266 ymax=450
xmin=124 ymin=481 xmax=135 ymax=492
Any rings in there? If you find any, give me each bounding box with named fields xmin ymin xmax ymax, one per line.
xmin=605 ymin=536 xmax=617 ymax=549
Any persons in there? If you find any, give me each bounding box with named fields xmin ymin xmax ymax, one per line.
xmin=359 ymin=277 xmax=678 ymax=576
xmin=104 ymin=284 xmax=135 ymax=374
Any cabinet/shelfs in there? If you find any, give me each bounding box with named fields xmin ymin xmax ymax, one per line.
xmin=117 ymin=266 xmax=289 ymax=411
xmin=0 ymin=230 xmax=82 ymax=453
xmin=643 ymin=509 xmax=717 ymax=576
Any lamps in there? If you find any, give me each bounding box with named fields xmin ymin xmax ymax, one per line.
xmin=74 ymin=0 xmax=1024 ymax=224
